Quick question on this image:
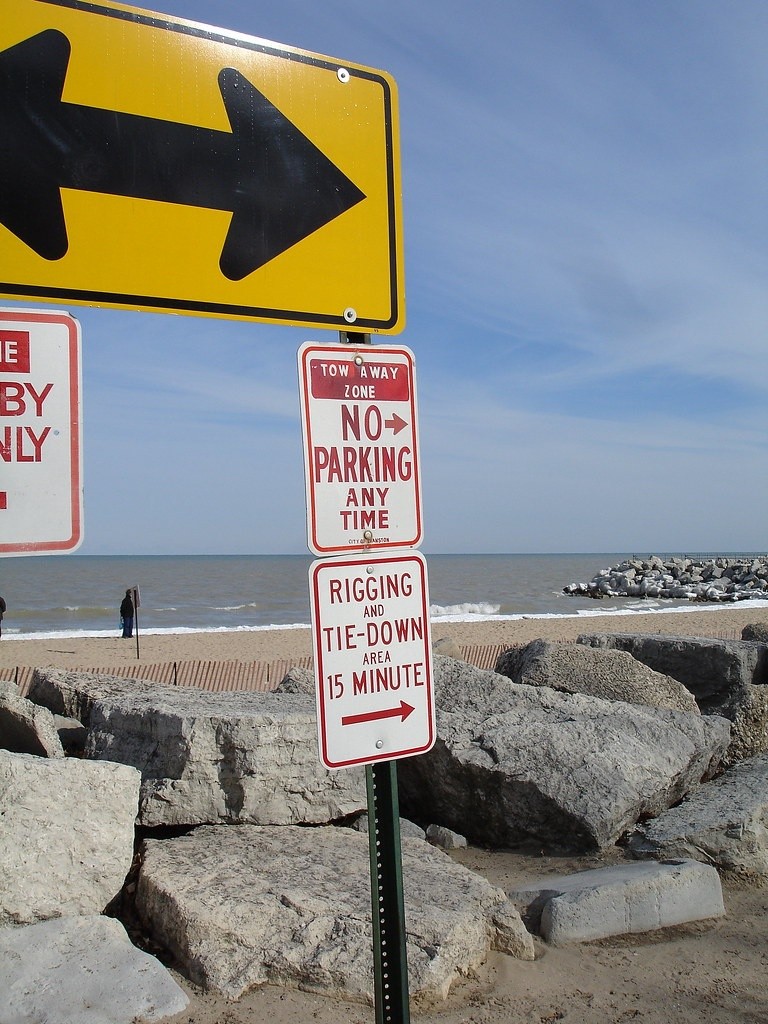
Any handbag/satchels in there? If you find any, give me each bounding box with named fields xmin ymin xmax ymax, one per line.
xmin=119 ymin=619 xmax=124 ymax=629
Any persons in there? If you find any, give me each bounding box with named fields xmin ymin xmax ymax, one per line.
xmin=120 ymin=589 xmax=134 ymax=638
xmin=0 ymin=597 xmax=6 ymax=638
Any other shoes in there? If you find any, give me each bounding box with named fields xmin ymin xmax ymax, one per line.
xmin=122 ymin=635 xmax=133 ymax=638
